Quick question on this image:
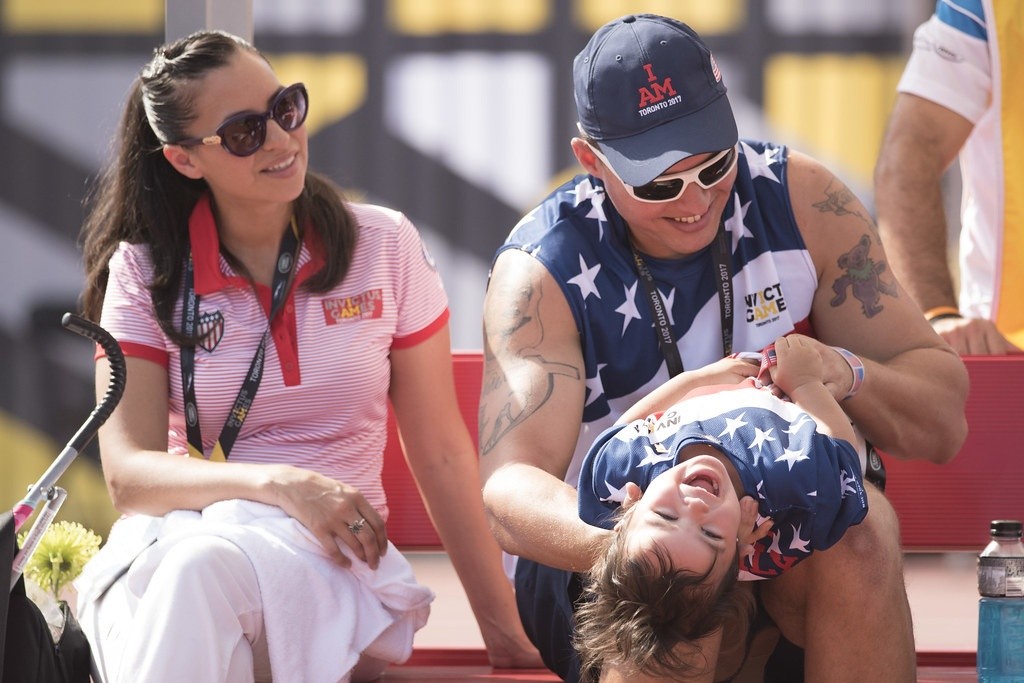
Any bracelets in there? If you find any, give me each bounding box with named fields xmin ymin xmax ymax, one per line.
xmin=829 ymin=346 xmax=865 ymax=401
xmin=923 ymin=306 xmax=962 ymax=321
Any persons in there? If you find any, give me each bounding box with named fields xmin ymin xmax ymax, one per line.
xmin=77 ymin=28 xmax=547 ymax=683
xmin=478 ymin=16 xmax=968 ymax=683
xmin=875 ymin=0 xmax=1024 ymax=356
xmin=577 ymin=336 xmax=870 ymax=683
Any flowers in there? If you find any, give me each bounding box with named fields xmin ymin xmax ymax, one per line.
xmin=18 ymin=521 xmax=102 ymax=599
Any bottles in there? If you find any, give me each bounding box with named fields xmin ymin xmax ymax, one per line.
xmin=976 ymin=520 xmax=1024 ymax=683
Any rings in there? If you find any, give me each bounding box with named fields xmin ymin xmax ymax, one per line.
xmin=349 ymin=518 xmax=365 ymax=534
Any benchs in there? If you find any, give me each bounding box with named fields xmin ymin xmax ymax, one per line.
xmin=379 ymin=357 xmax=1024 ymax=683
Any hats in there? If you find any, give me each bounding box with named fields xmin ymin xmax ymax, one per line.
xmin=572 ymin=13 xmax=738 ymax=186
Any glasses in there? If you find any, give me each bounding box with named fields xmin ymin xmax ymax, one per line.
xmin=582 ymin=139 xmax=738 ymax=203
xmin=166 ymin=82 xmax=309 ymax=158
